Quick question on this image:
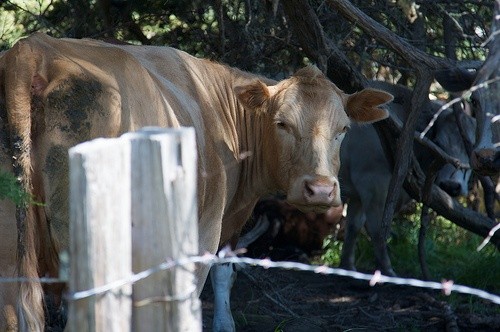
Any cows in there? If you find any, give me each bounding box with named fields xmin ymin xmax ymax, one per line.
xmin=1 ymin=32 xmax=394 ymax=332
xmin=338 ymin=80 xmax=494 ymax=278
